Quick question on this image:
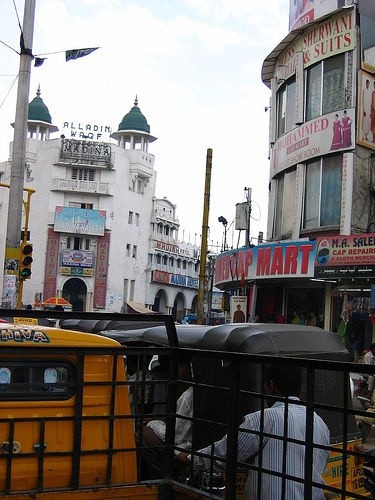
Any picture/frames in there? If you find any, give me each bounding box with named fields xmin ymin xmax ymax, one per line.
xmin=357 ymin=70 xmax=375 ymax=150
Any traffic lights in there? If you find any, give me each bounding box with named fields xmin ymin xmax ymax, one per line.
xmin=18 ymin=243 xmax=33 ymax=280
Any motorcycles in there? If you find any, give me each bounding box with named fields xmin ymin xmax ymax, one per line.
xmin=0 ymin=311 xmax=375 ymax=500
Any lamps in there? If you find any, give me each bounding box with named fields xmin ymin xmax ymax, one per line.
xmin=218 ymin=215 xmax=229 ymax=251
xmin=264 ymin=106 xmax=271 ymax=112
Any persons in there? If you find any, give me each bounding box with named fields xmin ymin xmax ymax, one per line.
xmin=138 ymin=356 xmax=194 ymax=455
xmin=361 ymin=343 xmax=375 ymax=397
xmin=177 ymin=360 xmax=330 ymax=500
xmin=233 ymin=305 xmax=245 ymax=323
xmin=248 ymin=311 xmax=284 ymax=324
xmin=290 ymin=310 xmax=324 ymax=327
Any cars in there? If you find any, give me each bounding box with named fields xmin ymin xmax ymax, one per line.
xmin=181 ymin=313 xmax=197 ymax=324
xmin=203 ymin=312 xmax=226 ymax=326
